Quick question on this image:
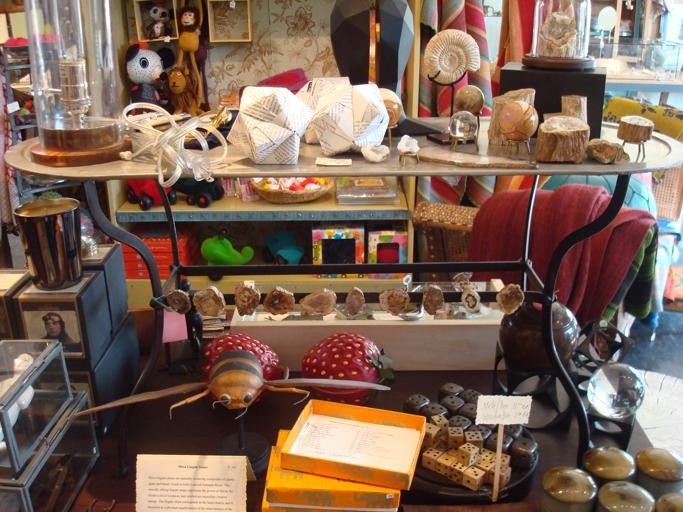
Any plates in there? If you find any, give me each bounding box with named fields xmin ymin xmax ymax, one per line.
xmin=416 ymin=416 xmax=538 ymax=500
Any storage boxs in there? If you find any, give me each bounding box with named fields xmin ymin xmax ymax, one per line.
xmin=1 ymin=244 xmax=141 ymax=512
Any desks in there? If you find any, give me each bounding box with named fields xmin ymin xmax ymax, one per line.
xmin=1 ymin=136 xmax=682 ymax=468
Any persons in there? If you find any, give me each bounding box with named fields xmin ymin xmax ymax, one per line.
xmin=33 ymin=314 xmax=80 ymax=354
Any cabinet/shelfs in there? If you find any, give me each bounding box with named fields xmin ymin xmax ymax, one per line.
xmin=587 ymin=37 xmax=683 ymax=125
xmin=132 ymin=0 xmax=253 ymax=43
xmin=103 ymin=175 xmax=416 ymax=280
xmin=0 ymin=45 xmax=38 ymax=145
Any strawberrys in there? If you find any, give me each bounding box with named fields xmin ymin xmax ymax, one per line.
xmin=190 ymin=331 xmax=280 ymax=406
xmin=301 ymin=332 xmax=398 ymax=404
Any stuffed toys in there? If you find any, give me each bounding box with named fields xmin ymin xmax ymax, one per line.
xmin=125 ymin=45 xmax=175 ymax=114
xmin=178 ymin=1 xmax=203 ymax=107
xmin=167 ymin=68 xmax=205 ymax=119
xmin=143 ymin=4 xmax=172 ymax=44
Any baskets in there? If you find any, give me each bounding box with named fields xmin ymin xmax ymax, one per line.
xmin=251 ymin=182 xmax=333 ymax=204
